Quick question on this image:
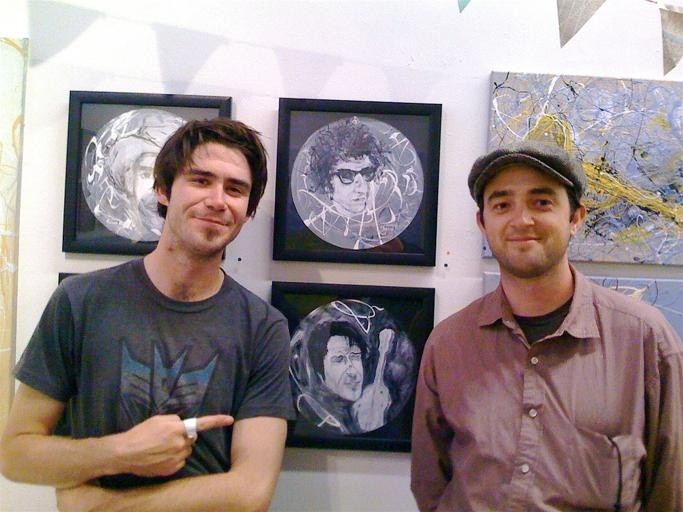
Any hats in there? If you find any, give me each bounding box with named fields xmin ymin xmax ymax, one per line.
xmin=465 ymin=142 xmax=587 ymax=206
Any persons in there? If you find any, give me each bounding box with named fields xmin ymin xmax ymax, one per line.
xmin=298 ymin=318 xmax=369 ymax=428
xmin=302 ymin=120 xmax=387 ymax=216
xmin=407 ymin=143 xmax=683 ymax=512
xmin=2 ymin=115 xmax=294 ymax=510
xmin=103 ymin=132 xmax=162 ymax=222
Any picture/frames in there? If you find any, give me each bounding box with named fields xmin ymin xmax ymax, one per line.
xmin=272 ymin=280 xmax=436 ymax=453
xmin=272 ymin=96 xmax=443 ymax=267
xmin=61 ymin=89 xmax=234 ymax=261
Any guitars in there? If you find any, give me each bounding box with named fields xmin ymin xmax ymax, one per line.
xmin=351 ymin=326 xmax=395 ymax=433
